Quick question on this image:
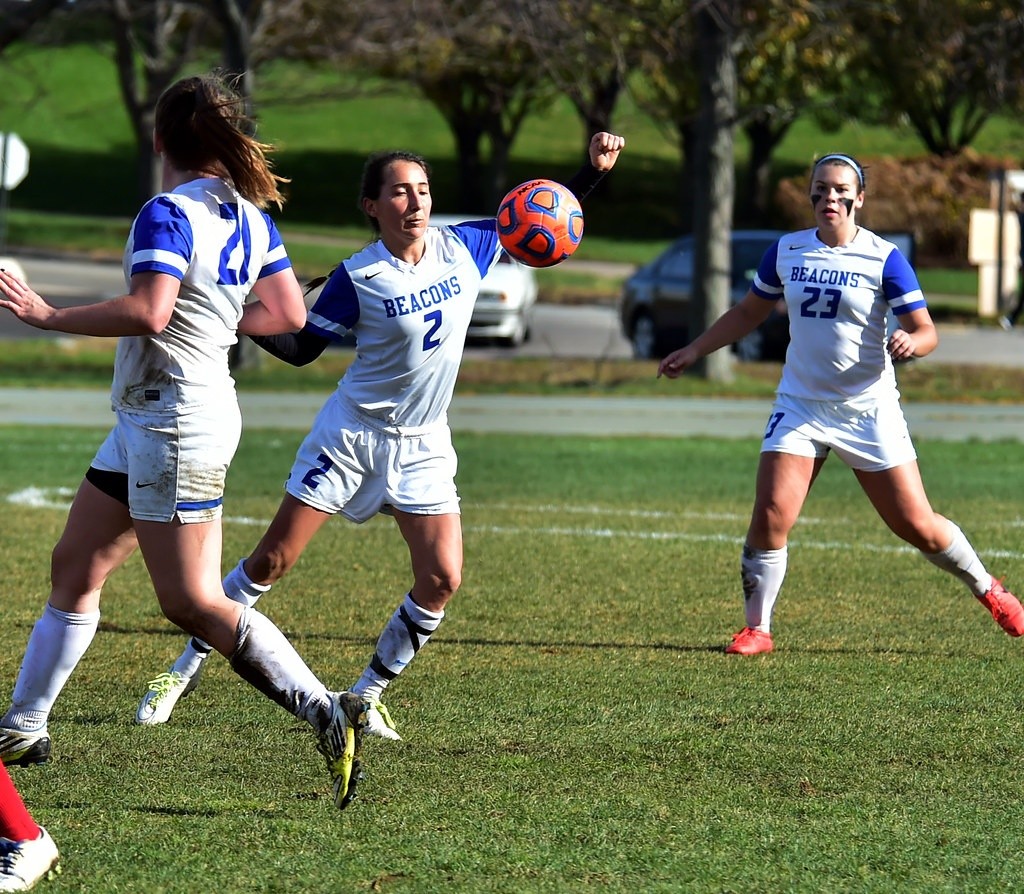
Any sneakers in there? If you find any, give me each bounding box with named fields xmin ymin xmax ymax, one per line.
xmin=724 ymin=627 xmax=774 ymax=655
xmin=0 ymin=826 xmax=63 ymax=894
xmin=134 ymin=663 xmax=199 ymax=725
xmin=0 ymin=722 xmax=52 ymax=768
xmin=315 ymin=690 xmax=371 ymax=811
xmin=972 ymin=575 xmax=1024 ymax=637
xmin=343 ymin=683 xmax=404 ymax=742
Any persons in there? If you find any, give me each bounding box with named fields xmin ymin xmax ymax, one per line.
xmin=136 ymin=132 xmax=624 ymax=744
xmin=0 ymin=77 xmax=371 ymax=812
xmin=656 ymin=153 xmax=1024 ymax=656
xmin=0 ymin=759 xmax=61 ymax=894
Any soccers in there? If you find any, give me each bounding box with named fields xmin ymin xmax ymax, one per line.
xmin=494 ymin=176 xmax=585 ymax=270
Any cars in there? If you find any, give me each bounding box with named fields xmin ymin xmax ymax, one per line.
xmin=618 ymin=227 xmax=914 ymax=365
xmin=428 ymin=212 xmax=536 ymax=346
